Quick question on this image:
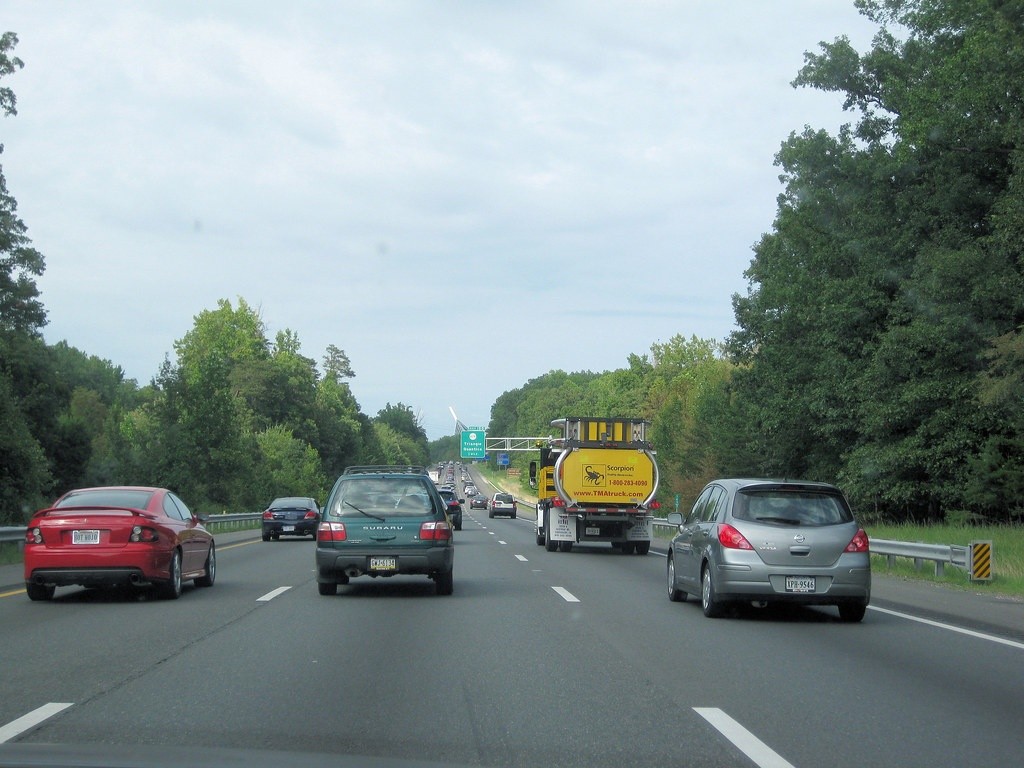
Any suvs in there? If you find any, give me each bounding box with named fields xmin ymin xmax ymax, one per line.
xmin=436 ymin=489 xmax=465 ymax=531
xmin=315 ymin=464 xmax=461 ymax=596
xmin=489 ymin=493 xmax=518 ymax=519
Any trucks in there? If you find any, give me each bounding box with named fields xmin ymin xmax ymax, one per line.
xmin=528 ymin=416 xmax=662 ymax=556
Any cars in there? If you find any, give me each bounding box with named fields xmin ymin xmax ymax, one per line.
xmin=24 ymin=485 xmax=216 ymax=602
xmin=470 ymin=495 xmax=488 ymax=510
xmin=466 ymin=489 xmax=477 ymax=498
xmin=465 ymin=486 xmax=475 ymax=494
xmin=667 ymin=479 xmax=870 ymax=622
xmin=429 ymin=460 xmax=474 ymax=492
xmin=262 ymin=497 xmax=321 ymax=542
